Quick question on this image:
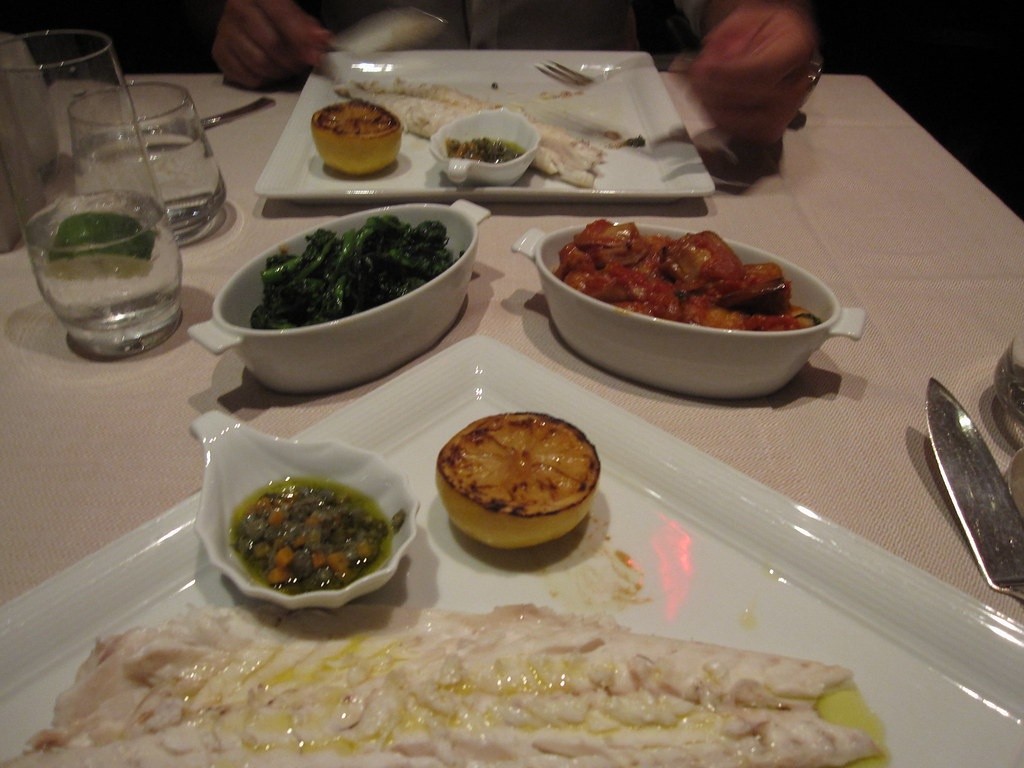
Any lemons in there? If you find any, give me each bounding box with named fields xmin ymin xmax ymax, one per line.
xmin=309 ymin=98 xmax=404 ymax=176
xmin=435 ymin=411 xmax=600 ymax=550
xmin=42 ymin=211 xmax=157 ymax=279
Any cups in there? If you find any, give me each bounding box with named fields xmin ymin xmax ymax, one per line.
xmin=995 ymin=331 xmax=1024 ymax=431
xmin=0 ymin=28 xmax=182 ymax=359
xmin=68 ymin=81 xmax=228 ymax=242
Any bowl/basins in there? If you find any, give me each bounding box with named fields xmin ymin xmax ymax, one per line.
xmin=511 ymin=222 xmax=864 ymax=401
xmin=188 ymin=410 xmax=421 ymax=610
xmin=187 ymin=199 xmax=493 ymax=395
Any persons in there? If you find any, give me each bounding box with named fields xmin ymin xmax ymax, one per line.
xmin=211 ymin=0 xmax=822 ymax=143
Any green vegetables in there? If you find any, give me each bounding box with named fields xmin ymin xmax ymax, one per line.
xmin=250 ymin=216 xmax=465 ymax=327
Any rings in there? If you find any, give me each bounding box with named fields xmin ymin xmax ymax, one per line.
xmin=809 ymin=61 xmax=822 ymax=85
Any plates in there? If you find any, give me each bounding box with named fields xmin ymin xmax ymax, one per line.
xmin=0 ymin=334 xmax=1024 ymax=768
xmin=253 ymin=48 xmax=716 ymax=204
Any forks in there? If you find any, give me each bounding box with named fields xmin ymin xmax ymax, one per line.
xmin=536 ymin=50 xmax=697 ymax=89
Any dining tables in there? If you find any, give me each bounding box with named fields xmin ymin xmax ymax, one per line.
xmin=1 ymin=55 xmax=1023 ymax=768
